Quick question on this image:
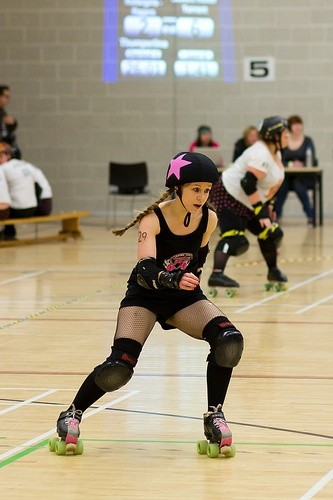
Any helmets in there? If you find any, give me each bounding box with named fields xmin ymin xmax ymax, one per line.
xmin=258 ymin=116 xmax=290 ymax=142
xmin=165 ymin=152 xmax=221 ymax=186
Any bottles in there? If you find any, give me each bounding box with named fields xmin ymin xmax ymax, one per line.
xmin=306 ymin=144 xmax=312 ymax=167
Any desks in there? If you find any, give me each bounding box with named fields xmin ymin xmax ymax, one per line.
xmin=216 ymin=166 xmax=324 ymax=227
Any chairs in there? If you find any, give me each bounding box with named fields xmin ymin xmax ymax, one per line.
xmin=106 ymin=162 xmax=150 ymax=230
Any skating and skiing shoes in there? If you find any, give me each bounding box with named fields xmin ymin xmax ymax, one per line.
xmin=49 ymin=404 xmax=83 ymax=454
xmin=206 ymin=270 xmax=240 ymax=299
xmin=197 ymin=404 xmax=236 ymax=457
xmin=264 ymin=267 xmax=289 ymax=294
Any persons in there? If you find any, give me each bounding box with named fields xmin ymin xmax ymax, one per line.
xmin=232 ymin=126 xmax=259 ymax=161
xmin=207 ymin=116 xmax=290 ymax=287
xmin=0 ymin=86 xmax=53 ymax=240
xmin=275 ymin=115 xmax=319 ymax=224
xmin=189 ymin=126 xmax=225 ymax=169
xmin=47 ymin=152 xmax=244 ymax=459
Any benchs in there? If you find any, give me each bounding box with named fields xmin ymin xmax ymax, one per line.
xmin=0 ymin=211 xmax=91 ymax=247
